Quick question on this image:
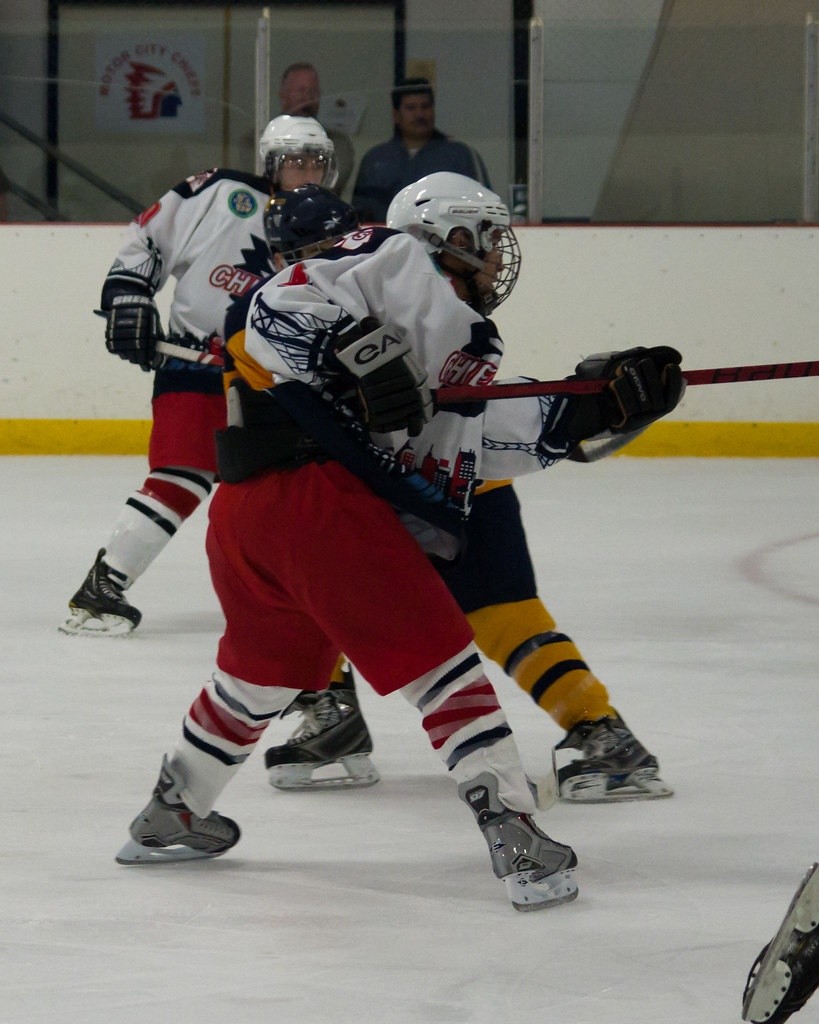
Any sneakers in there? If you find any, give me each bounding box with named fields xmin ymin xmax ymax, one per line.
xmin=264 ymin=665 xmax=380 ymax=790
xmin=58 ymin=548 xmax=143 ymax=638
xmin=525 ymin=713 xmax=674 ymax=809
xmin=455 ymin=773 xmax=578 ymax=911
xmin=116 ymin=753 xmax=242 ymax=864
xmin=742 ymin=858 xmax=818 ymax=1024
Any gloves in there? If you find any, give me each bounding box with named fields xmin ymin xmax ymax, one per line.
xmin=105 ymin=292 xmax=167 ymax=371
xmin=567 ymin=345 xmax=682 ymax=432
xmin=343 ymin=322 xmax=432 ymax=454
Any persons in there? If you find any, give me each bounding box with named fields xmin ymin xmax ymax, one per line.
xmin=235 ymin=61 xmax=354 ymax=198
xmin=219 ymin=186 xmax=676 ymax=804
xmin=53 ymin=113 xmax=341 ymax=634
xmin=348 ymin=78 xmax=494 ymax=233
xmin=106 ymin=170 xmax=584 ymax=915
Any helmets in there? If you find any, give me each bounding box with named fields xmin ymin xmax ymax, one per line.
xmin=259 ymin=113 xmax=334 ymax=195
xmin=261 ymin=182 xmax=362 ymax=272
xmin=386 ymin=171 xmax=522 ymax=319
